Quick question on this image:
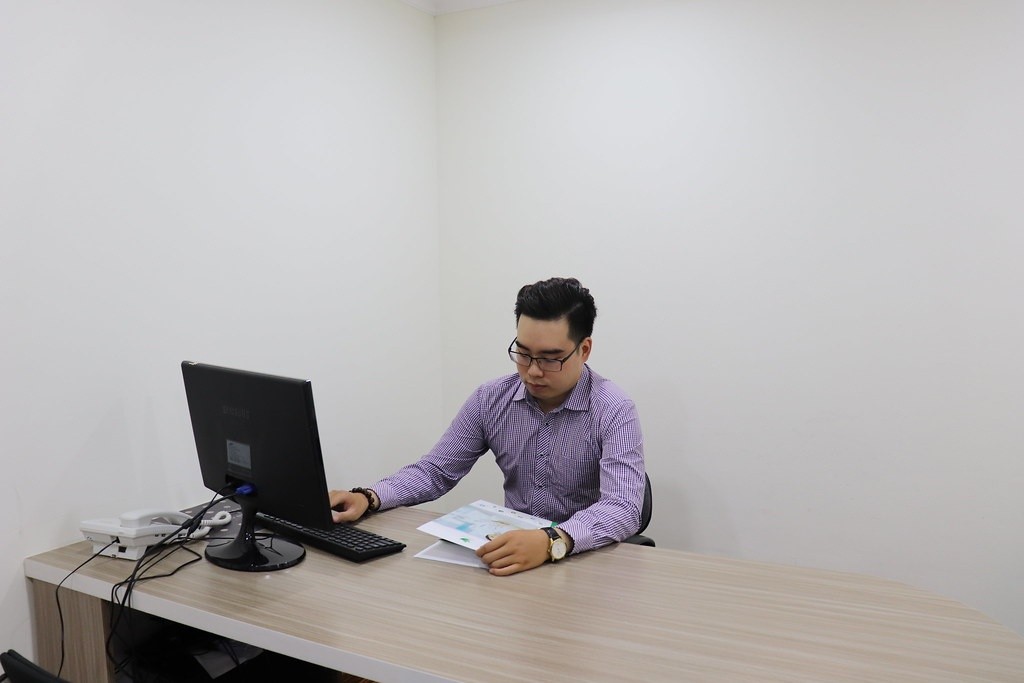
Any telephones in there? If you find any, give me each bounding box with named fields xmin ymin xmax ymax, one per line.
xmin=78 ymin=508 xmax=199 ymax=562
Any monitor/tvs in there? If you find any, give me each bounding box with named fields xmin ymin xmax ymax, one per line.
xmin=181 ymin=360 xmax=335 ymax=572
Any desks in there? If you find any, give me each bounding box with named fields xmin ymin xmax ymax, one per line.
xmin=24 ymin=504 xmax=1023 ymax=683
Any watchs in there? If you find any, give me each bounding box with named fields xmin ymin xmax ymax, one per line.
xmin=540 ymin=528 xmax=569 ymax=562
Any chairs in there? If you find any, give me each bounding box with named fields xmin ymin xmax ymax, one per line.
xmin=623 ymin=473 xmax=655 ymax=547
xmin=0 ymin=649 xmax=73 ymax=683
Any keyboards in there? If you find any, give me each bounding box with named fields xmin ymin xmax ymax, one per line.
xmin=253 ymin=512 xmax=407 ymax=562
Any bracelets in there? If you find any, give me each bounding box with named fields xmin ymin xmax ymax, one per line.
xmin=348 ymin=487 xmax=375 ymax=516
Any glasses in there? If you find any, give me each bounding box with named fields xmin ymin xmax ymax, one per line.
xmin=508 ymin=337 xmax=581 ymax=372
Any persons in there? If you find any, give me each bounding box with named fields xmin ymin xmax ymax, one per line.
xmin=326 ymin=277 xmax=647 ymax=576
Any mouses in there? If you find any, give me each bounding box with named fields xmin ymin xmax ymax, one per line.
xmin=330 ymin=503 xmax=345 ymax=513
